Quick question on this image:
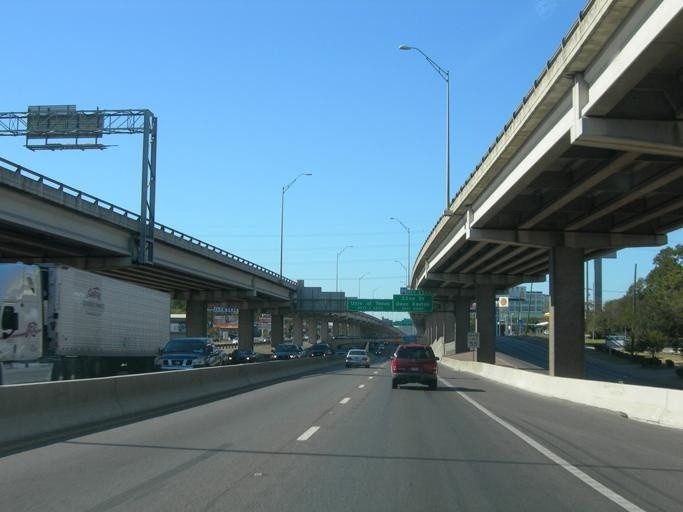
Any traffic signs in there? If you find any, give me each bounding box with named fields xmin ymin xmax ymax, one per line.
xmin=392 ymin=296 xmax=433 ymax=313
xmin=467 ymin=332 xmax=480 ymax=348
xmin=347 ymin=300 xmax=392 ymax=312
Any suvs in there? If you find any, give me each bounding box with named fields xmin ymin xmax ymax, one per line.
xmin=390 ymin=344 xmax=437 ymax=391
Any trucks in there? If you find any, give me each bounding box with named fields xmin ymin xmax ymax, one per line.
xmin=0 ymin=257 xmax=172 ymax=387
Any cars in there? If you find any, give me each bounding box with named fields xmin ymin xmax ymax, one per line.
xmin=153 ymin=338 xmax=334 ymax=372
xmin=375 ymin=341 xmax=388 ymax=356
xmin=344 ymin=349 xmax=371 ymax=368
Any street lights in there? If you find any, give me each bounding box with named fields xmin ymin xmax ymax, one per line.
xmin=333 ymin=215 xmax=412 ymax=294
xmin=399 ymin=44 xmax=453 ymax=214
xmin=278 ymin=172 xmax=313 ymax=284
